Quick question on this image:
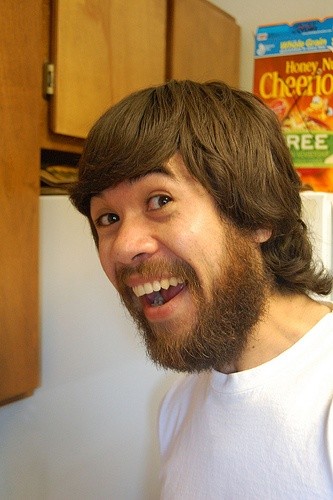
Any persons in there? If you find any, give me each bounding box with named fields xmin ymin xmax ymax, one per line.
xmin=67 ymin=80 xmax=333 ymax=499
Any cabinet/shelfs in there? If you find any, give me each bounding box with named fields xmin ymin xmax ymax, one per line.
xmin=42 ymin=0 xmax=239 ymax=146
